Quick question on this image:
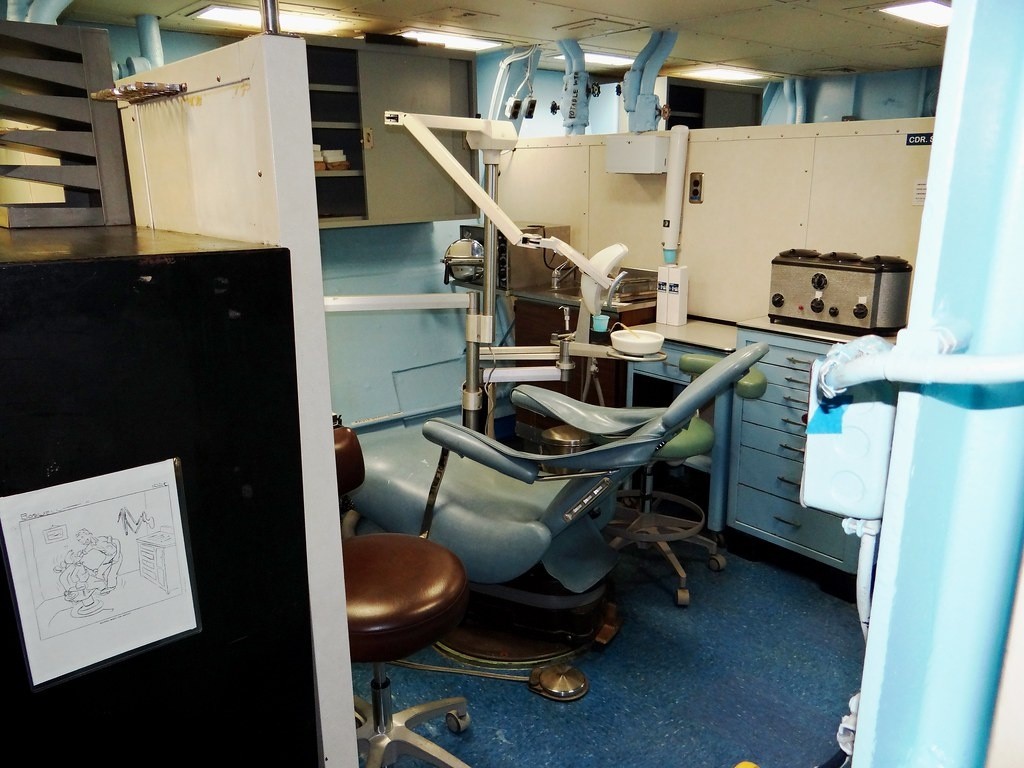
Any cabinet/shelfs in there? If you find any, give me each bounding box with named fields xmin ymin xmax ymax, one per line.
xmin=726 ymin=316 xmax=862 ymax=595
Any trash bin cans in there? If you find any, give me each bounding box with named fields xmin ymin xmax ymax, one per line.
xmin=540 ymin=425 xmax=595 ymax=476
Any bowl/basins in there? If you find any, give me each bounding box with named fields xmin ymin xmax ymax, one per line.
xmin=612 ymin=330 xmax=665 ymax=356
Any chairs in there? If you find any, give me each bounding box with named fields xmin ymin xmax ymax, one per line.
xmin=588 ymin=352 xmax=769 ymax=606
xmin=333 ymin=427 xmax=477 ymax=767
xmin=340 ymin=339 xmax=774 ymax=671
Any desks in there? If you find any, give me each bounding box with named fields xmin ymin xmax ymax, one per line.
xmin=625 ymin=318 xmax=737 ymax=545
